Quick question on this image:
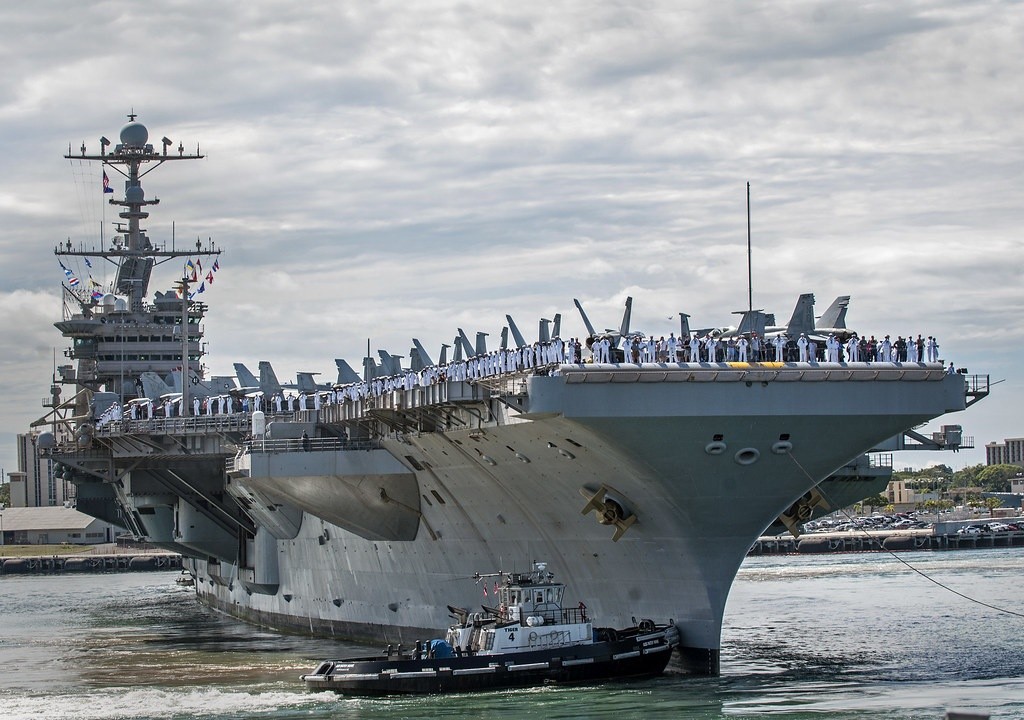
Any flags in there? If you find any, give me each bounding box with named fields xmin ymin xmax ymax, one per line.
xmin=493 ymin=581 xmax=498 ymax=594
xmin=176 ymin=258 xmax=219 ymax=299
xmin=58 ymin=257 xmax=103 ymax=299
xmin=103 ymin=169 xmax=113 ymax=193
xmin=483 ymin=583 xmax=488 ymax=596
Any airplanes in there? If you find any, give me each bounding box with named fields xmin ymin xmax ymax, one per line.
xmin=123 ymin=313 xmax=562 ymax=416
xmin=679 ymin=292 xmax=858 ymax=350
xmin=573 ymin=295 xmax=645 ymax=351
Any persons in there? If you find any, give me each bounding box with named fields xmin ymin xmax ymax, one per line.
xmin=130 ymin=400 xmax=156 ymax=420
xmin=622 ymin=332 xmax=940 ymax=363
xmin=96 ymin=402 xmax=123 ymax=430
xmin=568 ymin=338 xmax=581 ymax=364
xmin=203 ymin=396 xmax=213 ymax=415
xmin=314 ymin=389 xmax=321 ymax=410
xmin=193 ymin=396 xmax=200 ymax=415
xmin=591 ymin=336 xmax=617 ymax=363
xmin=286 ymin=393 xmax=296 ymax=410
xmin=242 ymin=394 xmax=266 ymax=413
xmin=227 ymin=396 xmax=238 ymax=414
xmin=327 ymin=336 xmax=565 ymax=404
xmin=178 ymin=397 xmax=184 ymax=416
xmin=218 ymin=395 xmax=225 ymax=414
xmin=162 ymin=397 xmax=175 ymax=418
xmin=271 ymin=393 xmax=282 ymax=411
xmin=298 ymin=391 xmax=308 ymax=410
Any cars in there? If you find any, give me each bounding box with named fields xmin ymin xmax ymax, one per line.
xmin=802 ymin=509 xmax=952 ymax=534
xmin=957 ymin=521 xmax=1024 ymax=534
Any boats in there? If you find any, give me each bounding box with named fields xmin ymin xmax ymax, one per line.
xmin=298 ymin=555 xmax=682 ymax=697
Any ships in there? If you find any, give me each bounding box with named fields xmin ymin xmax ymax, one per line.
xmin=27 ymin=108 xmax=990 ymax=679
